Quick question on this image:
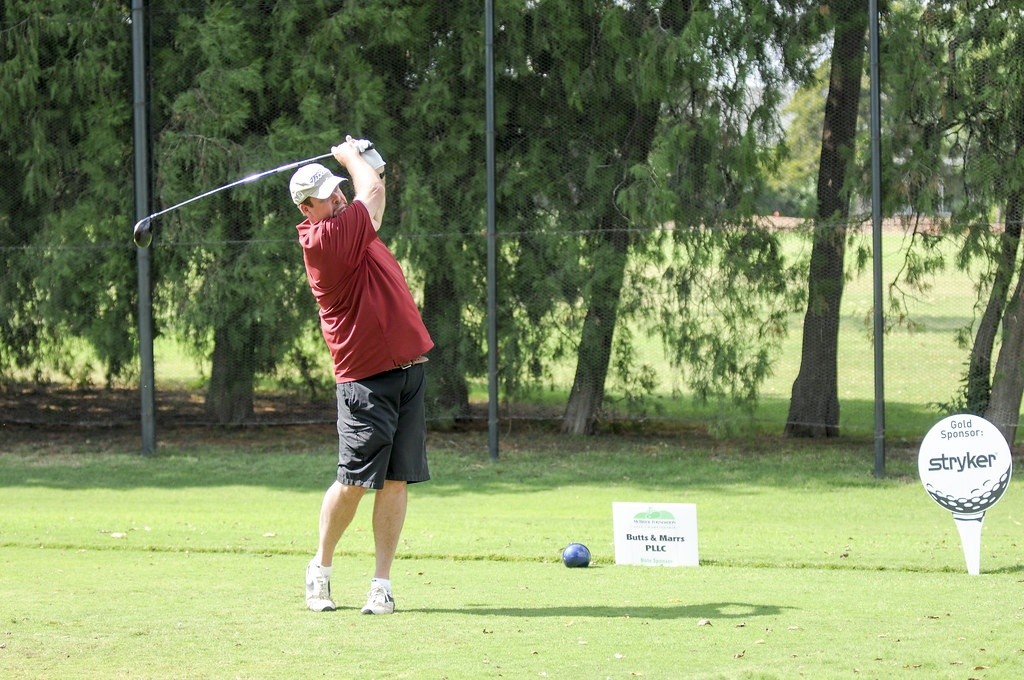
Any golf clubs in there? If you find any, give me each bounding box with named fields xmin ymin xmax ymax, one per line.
xmin=132 ymin=142 xmax=374 ymax=249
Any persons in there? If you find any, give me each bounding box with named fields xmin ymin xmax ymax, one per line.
xmin=289 ymin=135 xmax=434 ymax=615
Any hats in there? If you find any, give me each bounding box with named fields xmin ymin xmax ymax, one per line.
xmin=289 ymin=163 xmax=348 ymax=205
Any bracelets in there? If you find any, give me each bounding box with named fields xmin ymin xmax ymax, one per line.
xmin=379 ymin=171 xmax=386 ymax=178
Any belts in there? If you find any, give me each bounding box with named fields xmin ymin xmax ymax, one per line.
xmin=386 ymin=355 xmax=429 ymax=372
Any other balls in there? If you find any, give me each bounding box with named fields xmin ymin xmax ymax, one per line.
xmin=563 ymin=543 xmax=591 ymax=568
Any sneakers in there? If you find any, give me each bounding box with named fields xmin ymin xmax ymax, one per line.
xmin=305 ymin=560 xmax=336 ymax=612
xmin=360 ymin=586 xmax=395 ymax=615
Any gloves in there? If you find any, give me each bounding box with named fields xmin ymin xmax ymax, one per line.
xmin=346 ymin=135 xmax=386 ymax=170
